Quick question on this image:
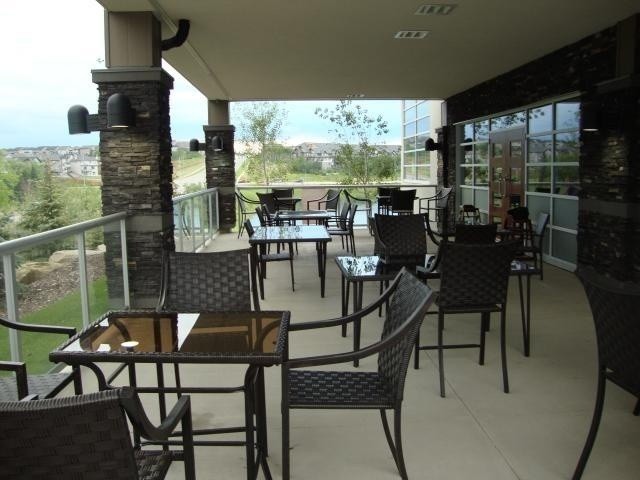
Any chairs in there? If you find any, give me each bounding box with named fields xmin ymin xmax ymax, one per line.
xmin=329 ymin=202 xmax=351 ymax=252
xmin=243 ymin=219 xmax=295 ymax=292
xmin=155 ymin=246 xmax=262 ymax=451
xmin=0 ymin=386 xmax=196 ymax=480
xmin=459 ymin=205 xmax=482 ymax=226
xmin=261 ymin=203 xmax=284 ymax=226
xmin=512 ymin=212 xmax=550 ymax=280
xmin=455 ymin=223 xmax=497 ymax=243
xmin=571 ymin=263 xmax=640 ymax=480
xmin=323 ymin=204 xmax=358 ymax=256
xmin=508 ymin=207 xmax=534 ymax=246
xmin=255 ymin=207 xmax=284 ymax=255
xmin=307 ymin=189 xmax=343 ymax=226
xmin=273 ymin=196 xmax=302 ymax=226
xmin=344 ymin=189 xmax=372 ymax=230
xmin=373 ymin=213 xmax=427 ymax=318
xmin=377 ymin=188 xmax=400 ymax=215
xmin=282 ymin=267 xmax=437 ymax=480
xmin=256 ymin=192 xmax=280 ymax=226
xmin=0 ymin=317 xmax=83 ymax=402
xmin=391 ymin=189 xmax=416 ymax=215
xmin=414 ymin=238 xmax=513 ymax=398
xmin=272 ymin=186 xmax=293 ymax=224
xmin=419 ymin=187 xmax=453 ymax=236
xmin=235 ymin=189 xmax=265 ymax=239
